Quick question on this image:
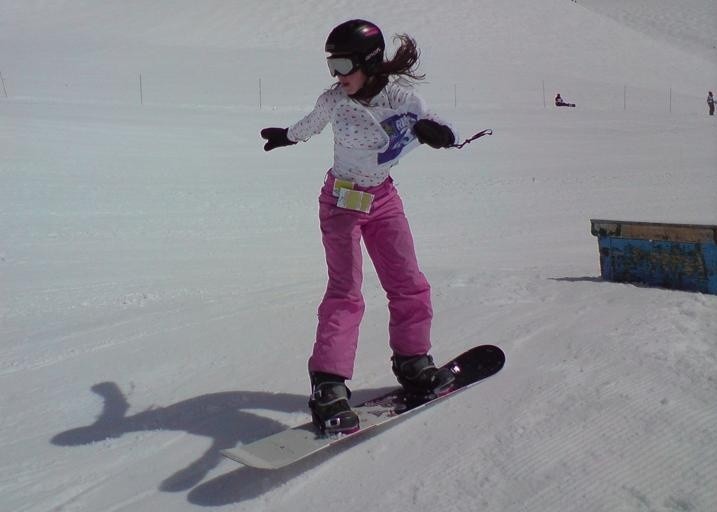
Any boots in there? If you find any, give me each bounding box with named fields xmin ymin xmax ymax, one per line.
xmin=390 ymin=354 xmax=456 ymax=392
xmin=308 ymin=376 xmax=361 ymax=436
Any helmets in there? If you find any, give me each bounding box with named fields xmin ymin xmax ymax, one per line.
xmin=323 ymin=19 xmax=388 ymax=64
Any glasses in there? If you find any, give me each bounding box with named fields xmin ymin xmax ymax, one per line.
xmin=325 ymin=54 xmax=360 ymax=78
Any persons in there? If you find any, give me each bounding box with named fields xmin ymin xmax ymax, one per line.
xmin=260 ymin=17 xmax=471 ymax=437
xmin=707 ymin=90 xmax=716 ymax=116
xmin=553 ymin=92 xmax=576 ymax=109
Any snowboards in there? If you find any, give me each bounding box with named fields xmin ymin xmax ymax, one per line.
xmin=218 ymin=345 xmax=505 ymax=472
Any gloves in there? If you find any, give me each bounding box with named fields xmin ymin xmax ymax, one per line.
xmin=410 ymin=121 xmax=454 ymax=149
xmin=259 ymin=126 xmax=294 ymax=152
